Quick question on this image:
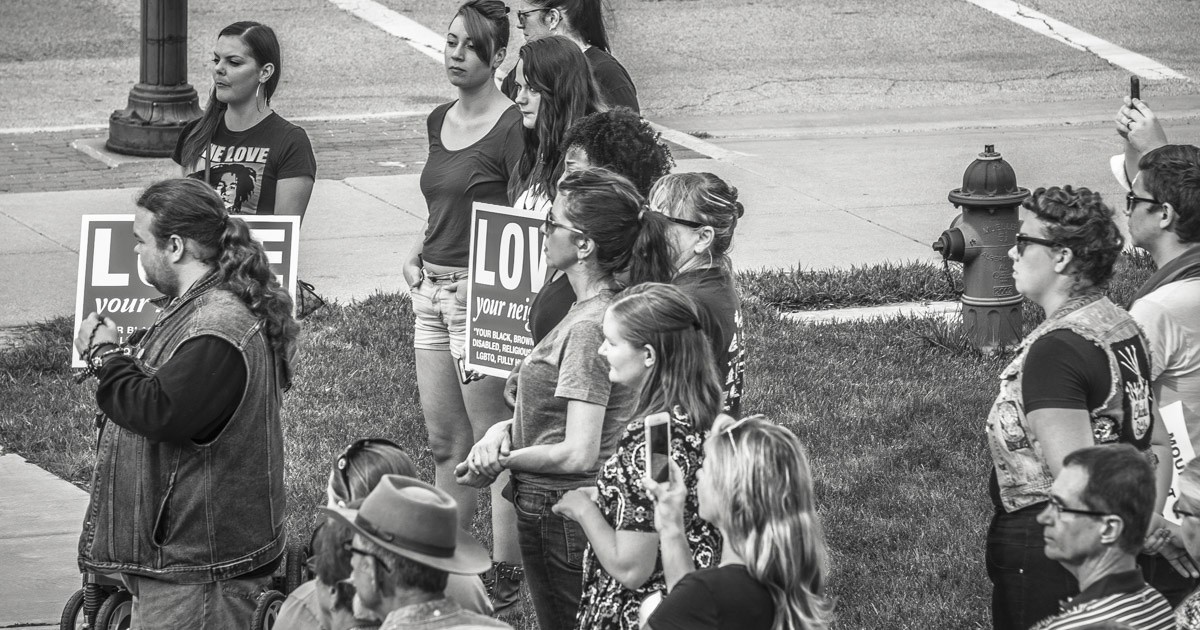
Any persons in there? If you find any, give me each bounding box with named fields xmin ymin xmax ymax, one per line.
xmin=1110 ymin=75 xmax=1200 ymax=610
xmin=640 ymin=412 xmax=837 ymax=630
xmin=170 ymin=21 xmax=315 ymax=231
xmin=403 ymin=0 xmax=531 ymax=620
xmin=984 ymin=187 xmax=1156 ymax=630
xmin=553 ymin=283 xmax=726 ymax=630
xmin=507 ymin=35 xmax=601 ymax=214
xmin=268 ymin=438 xmax=518 ymax=630
xmin=501 ymin=0 xmax=642 ymax=120
xmin=75 ymin=179 xmax=301 ymax=630
xmin=1028 ymin=443 xmax=1176 ymax=630
xmin=1173 ymin=456 xmax=1200 ymax=630
xmin=647 ymin=171 xmax=745 ymax=420
xmin=453 ymin=169 xmax=679 ymax=630
xmin=527 ymin=107 xmax=677 ymax=349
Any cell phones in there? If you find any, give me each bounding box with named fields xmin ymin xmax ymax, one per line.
xmin=644 ymin=411 xmax=671 ymax=498
xmin=1130 ymin=75 xmax=1140 ymax=109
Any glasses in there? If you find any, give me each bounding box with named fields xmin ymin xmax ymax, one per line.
xmin=720 ymin=413 xmax=766 ymax=460
xmin=1049 ymin=492 xmax=1119 ymax=522
xmin=516 ymin=9 xmax=564 ymax=26
xmin=544 ymin=208 xmax=599 ymax=248
xmin=1013 ymin=231 xmax=1076 ymax=258
xmin=337 ymin=436 xmax=404 ymax=500
xmin=1126 ymin=191 xmax=1180 ymax=221
xmin=1172 ymin=498 xmax=1200 ymax=520
xmin=342 ymin=539 xmax=392 ymax=575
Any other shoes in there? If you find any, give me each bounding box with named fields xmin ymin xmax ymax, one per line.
xmin=484 ymin=562 xmax=525 ymax=622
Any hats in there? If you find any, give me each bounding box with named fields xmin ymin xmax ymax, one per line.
xmin=319 ymin=473 xmax=493 ymax=574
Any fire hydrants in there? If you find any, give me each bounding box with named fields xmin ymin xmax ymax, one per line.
xmin=933 ymin=145 xmax=1031 ymax=356
